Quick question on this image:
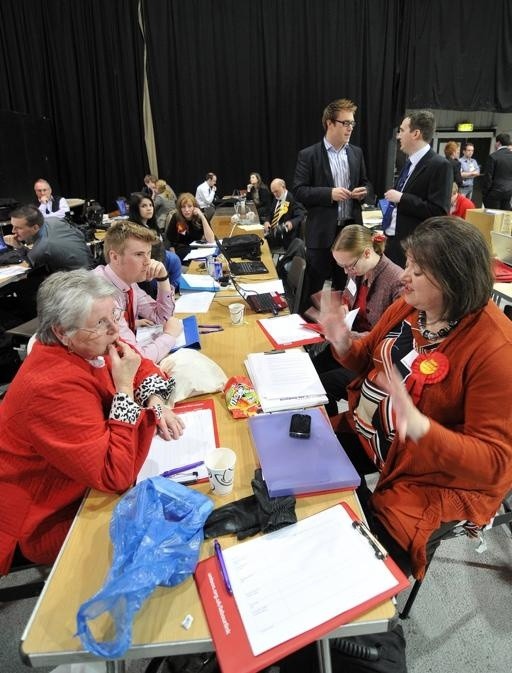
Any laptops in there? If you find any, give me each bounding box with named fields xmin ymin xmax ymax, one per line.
xmin=112 ymin=198 xmax=129 ymax=215
xmin=210 ymin=233 xmax=270 ymax=275
xmin=226 ymin=273 xmax=286 ymax=314
xmin=1 ymin=223 xmax=14 ymax=252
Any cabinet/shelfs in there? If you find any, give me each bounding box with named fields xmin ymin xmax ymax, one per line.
xmin=393 ymin=131 xmax=496 ymax=178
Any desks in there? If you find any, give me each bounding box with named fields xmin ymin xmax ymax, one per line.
xmin=0 ymin=229 xmax=107 ymax=289
xmin=20 ymin=300 xmax=401 ymax=670
xmin=175 ymin=201 xmax=280 ymax=299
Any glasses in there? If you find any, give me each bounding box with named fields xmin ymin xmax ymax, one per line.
xmin=67 ymin=306 xmax=122 ymax=336
xmin=332 ymin=118 xmax=357 ymax=128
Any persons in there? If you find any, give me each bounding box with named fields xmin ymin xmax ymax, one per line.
xmin=459 ymin=143 xmax=480 ymax=200
xmin=309 ymin=225 xmax=404 ymax=420
xmin=451 ymin=182 xmax=475 ymax=219
xmin=480 ymin=133 xmax=512 ymax=211
xmin=293 ymin=99 xmax=374 ymax=325
xmin=0 ymin=268 xmax=187 ymax=577
xmin=319 ymin=216 xmax=510 ymax=581
xmin=3 ymin=179 xmax=96 ymax=295
xmin=96 ymin=174 xmax=308 ymax=365
xmin=444 ymin=140 xmax=464 ymax=193
xmin=384 ymin=110 xmax=453 ymax=269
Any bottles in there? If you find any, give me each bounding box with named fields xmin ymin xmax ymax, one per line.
xmin=209 ymin=253 xmax=223 ymax=279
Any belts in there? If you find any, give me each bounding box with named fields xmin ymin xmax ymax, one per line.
xmin=459 ymin=184 xmax=470 ymax=189
xmin=336 ymin=217 xmax=354 ymax=229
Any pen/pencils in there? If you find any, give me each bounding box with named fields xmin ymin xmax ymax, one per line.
xmin=213 ymin=538 xmax=235 ymax=597
xmin=161 ymin=460 xmax=206 ymax=479
xmin=198 ymin=324 xmax=224 ymax=334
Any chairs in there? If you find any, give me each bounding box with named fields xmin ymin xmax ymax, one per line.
xmin=275 ymin=237 xmax=307 ymax=287
xmin=285 ymin=256 xmax=306 ymax=314
xmin=271 ymin=214 xmax=308 ymax=259
xmin=399 ymin=499 xmax=512 ymax=620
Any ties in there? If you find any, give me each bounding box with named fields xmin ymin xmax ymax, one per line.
xmin=271 ymin=198 xmax=282 ymax=231
xmin=123 ymin=287 xmax=137 ymax=334
xmin=209 ymin=188 xmax=211 ymax=195
xmin=380 ymin=158 xmax=415 ymax=231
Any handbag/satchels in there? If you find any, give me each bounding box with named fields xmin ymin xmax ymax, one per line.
xmin=143 ymin=621 xmax=409 ymax=673
xmin=214 ymin=197 xmax=239 ymax=207
xmin=220 ymin=234 xmax=263 ymax=261
xmin=80 ymin=198 xmax=105 ymax=226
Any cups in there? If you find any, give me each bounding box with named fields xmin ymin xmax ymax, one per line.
xmin=245 ymin=210 xmax=255 ymax=220
xmin=226 ymin=300 xmax=247 ymax=326
xmin=231 ymin=213 xmax=241 ymax=223
xmin=202 ymin=444 xmax=237 ymax=498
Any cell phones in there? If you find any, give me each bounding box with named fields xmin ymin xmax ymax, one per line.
xmin=288 ymin=410 xmax=312 ymax=438
